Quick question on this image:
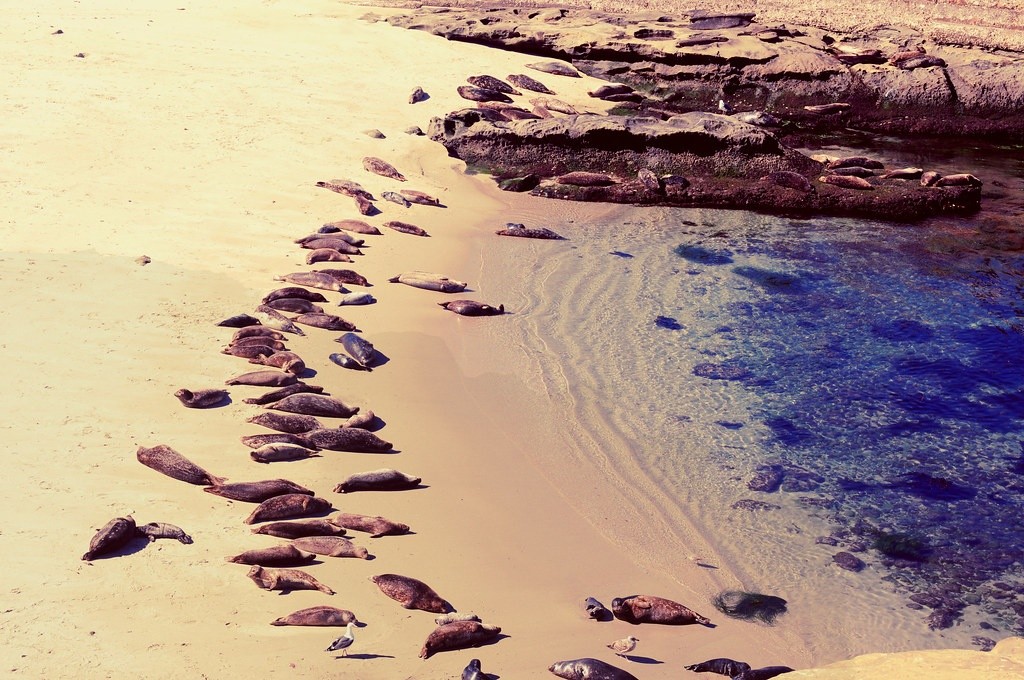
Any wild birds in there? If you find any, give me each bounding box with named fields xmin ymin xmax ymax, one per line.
xmin=609 ymin=636 xmax=639 ymax=661
xmin=326 ymin=622 xmax=356 ymax=658
